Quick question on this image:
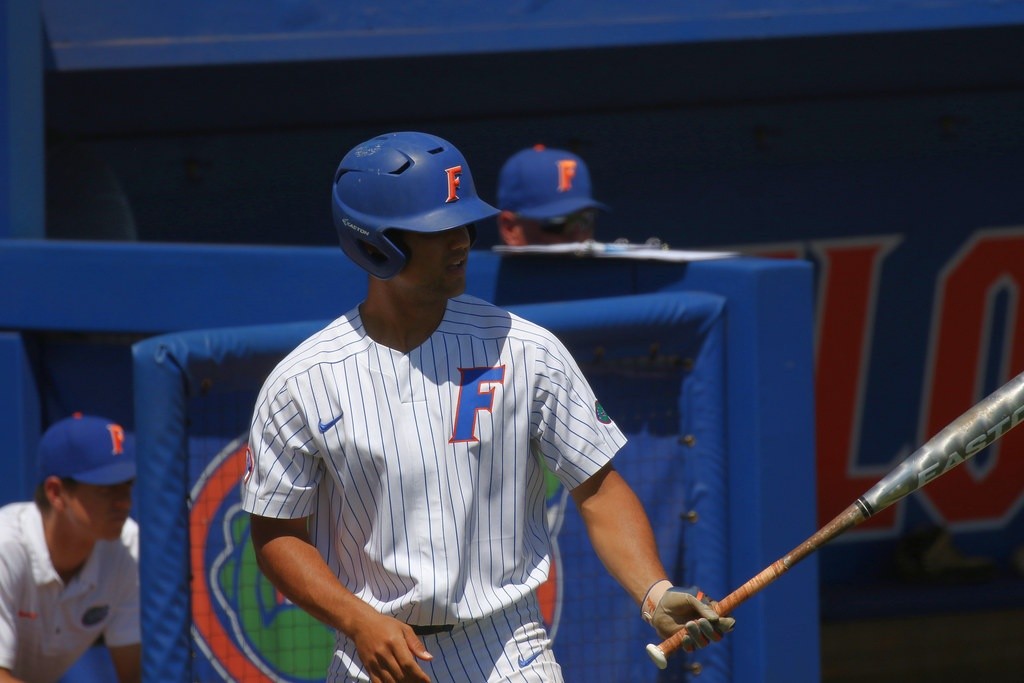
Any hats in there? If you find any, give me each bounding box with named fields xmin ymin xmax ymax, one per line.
xmin=498 ymin=145 xmax=612 ymax=222
xmin=36 ymin=412 xmax=138 ymax=486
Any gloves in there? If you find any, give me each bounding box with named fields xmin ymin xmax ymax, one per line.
xmin=640 ymin=579 xmax=735 ymax=654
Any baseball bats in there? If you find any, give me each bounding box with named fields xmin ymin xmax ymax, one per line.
xmin=645 ymin=371 xmax=1023 ymax=669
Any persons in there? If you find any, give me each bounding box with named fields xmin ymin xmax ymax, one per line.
xmin=497 ymin=144 xmax=612 ymax=246
xmin=240 ymin=133 xmax=736 ymax=683
xmin=0 ymin=412 xmax=142 ymax=683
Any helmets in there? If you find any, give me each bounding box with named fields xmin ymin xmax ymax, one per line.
xmin=332 ymin=131 xmax=501 ymax=280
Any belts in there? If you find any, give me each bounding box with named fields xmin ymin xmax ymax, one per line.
xmin=408 ymin=624 xmax=452 ymax=638
